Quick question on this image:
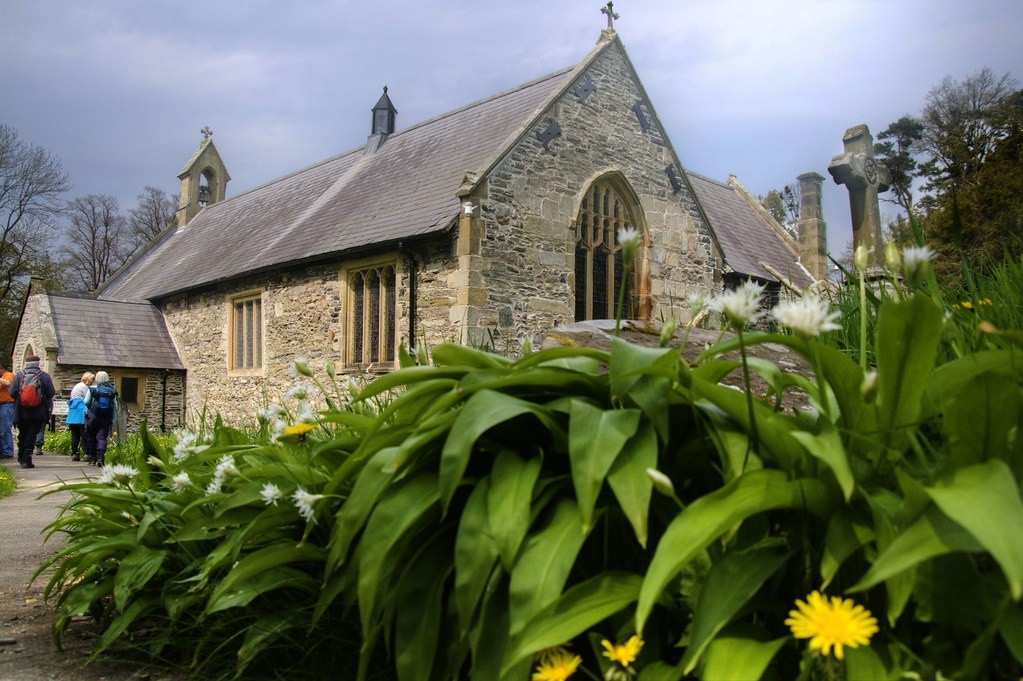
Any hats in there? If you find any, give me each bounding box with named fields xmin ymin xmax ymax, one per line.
xmin=26 ymin=356 xmax=40 ymax=362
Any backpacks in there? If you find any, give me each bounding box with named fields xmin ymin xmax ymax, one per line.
xmin=93 ymin=387 xmax=119 ymax=412
xmin=19 ymin=369 xmax=44 ymax=408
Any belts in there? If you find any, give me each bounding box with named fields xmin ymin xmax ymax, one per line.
xmin=0 ymin=402 xmax=15 ymax=405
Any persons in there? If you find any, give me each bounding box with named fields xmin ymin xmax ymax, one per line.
xmin=7 ymin=355 xmax=56 ymax=469
xmin=83 ymin=371 xmax=119 ymax=467
xmin=0 ymin=357 xmax=15 ymax=459
xmin=66 ymin=372 xmax=96 ymax=462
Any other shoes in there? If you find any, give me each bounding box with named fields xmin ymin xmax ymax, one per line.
xmin=72 ymin=452 xmax=80 ymax=461
xmin=0 ymin=454 xmax=14 ymax=459
xmin=35 ymin=446 xmax=43 ymax=455
xmin=89 ymin=458 xmax=96 ymax=465
xmin=97 ymin=458 xmax=102 ymax=467
xmin=21 ymin=461 xmax=34 ymax=468
xmin=84 ymin=454 xmax=89 ymax=461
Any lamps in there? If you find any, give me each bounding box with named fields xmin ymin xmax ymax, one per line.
xmin=460 ymin=201 xmax=478 ymax=218
